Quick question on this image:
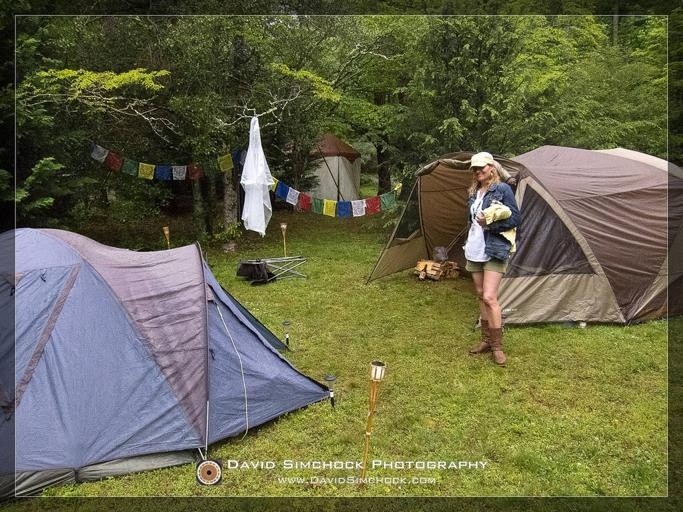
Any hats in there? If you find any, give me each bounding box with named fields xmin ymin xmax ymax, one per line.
xmin=470 ymin=152 xmax=494 ymax=170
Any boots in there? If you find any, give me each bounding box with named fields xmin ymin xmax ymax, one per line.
xmin=469 ymin=318 xmax=491 ymax=353
xmin=489 ymin=327 xmax=506 ymax=364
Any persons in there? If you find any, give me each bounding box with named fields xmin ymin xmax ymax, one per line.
xmin=463 ymin=152 xmax=522 ymax=367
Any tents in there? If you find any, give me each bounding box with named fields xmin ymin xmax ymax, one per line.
xmin=0 ymin=228 xmax=334 ymax=503
xmin=365 ymin=144 xmax=683 ymax=331
